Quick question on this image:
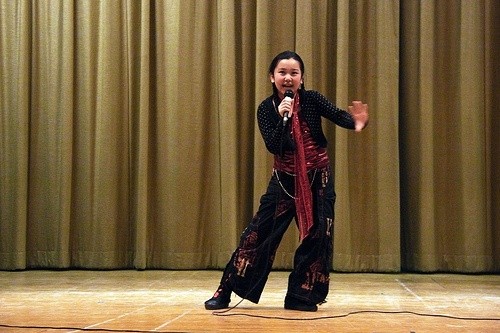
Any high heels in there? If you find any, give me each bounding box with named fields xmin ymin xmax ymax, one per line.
xmin=284 ymin=294 xmax=318 ymax=312
xmin=205 ymin=285 xmax=231 ymax=310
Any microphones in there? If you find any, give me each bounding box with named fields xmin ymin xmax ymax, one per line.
xmin=281 ymin=89 xmax=295 ymax=127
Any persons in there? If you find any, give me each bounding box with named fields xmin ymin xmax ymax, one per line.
xmin=205 ymin=51 xmax=369 ymax=312
